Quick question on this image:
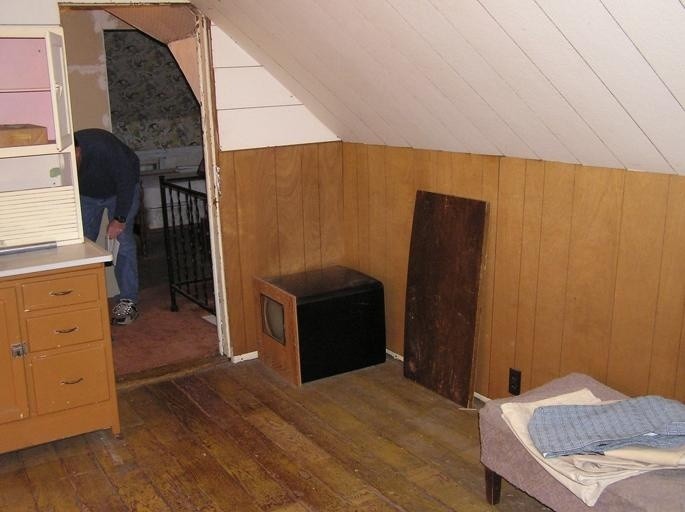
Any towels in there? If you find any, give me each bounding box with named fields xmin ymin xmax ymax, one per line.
xmin=497 ymin=382 xmax=682 ymax=506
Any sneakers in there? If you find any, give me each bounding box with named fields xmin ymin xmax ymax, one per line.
xmin=111 ymin=297 xmax=141 ymax=326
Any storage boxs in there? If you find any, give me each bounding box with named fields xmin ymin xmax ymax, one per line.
xmin=2 ymin=123 xmax=50 ymax=147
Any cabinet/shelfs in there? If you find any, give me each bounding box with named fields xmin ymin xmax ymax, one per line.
xmin=0 ymin=264 xmax=123 ymax=453
xmin=1 ymin=23 xmax=84 ymax=249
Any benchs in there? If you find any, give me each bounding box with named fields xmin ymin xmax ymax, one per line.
xmin=469 ymin=367 xmax=684 ymax=512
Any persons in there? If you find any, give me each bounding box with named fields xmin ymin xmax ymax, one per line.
xmin=73 ymin=127 xmax=143 ymax=326
